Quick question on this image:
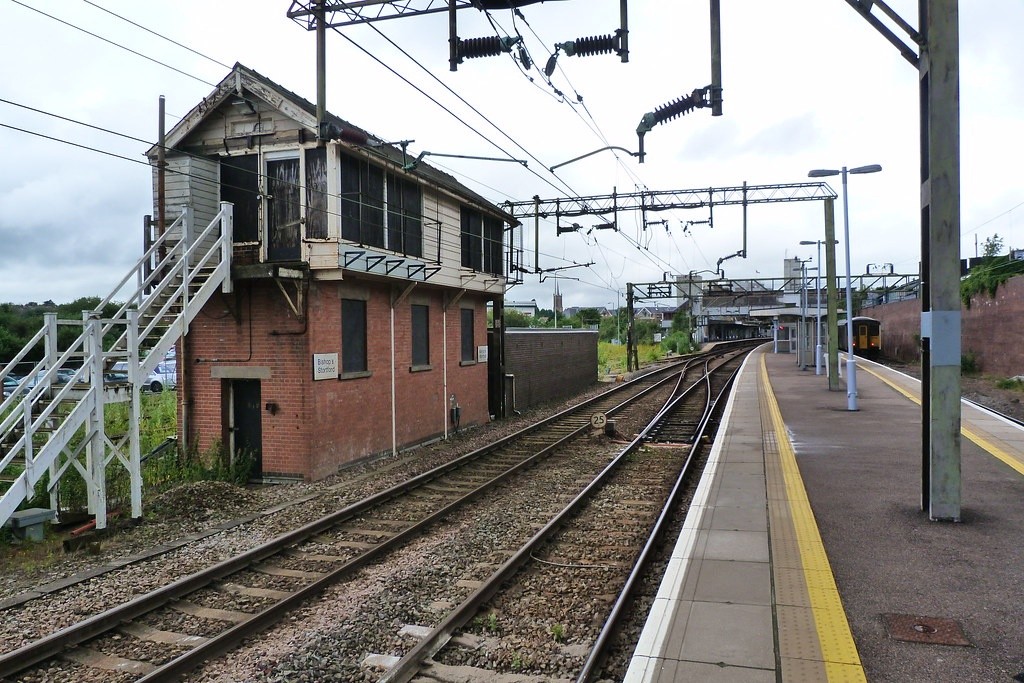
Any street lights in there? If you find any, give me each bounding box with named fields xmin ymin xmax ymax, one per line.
xmin=800 ymin=240 xmax=841 ymax=376
xmin=554 ymin=270 xmax=567 ymax=329
xmin=807 ymin=163 xmax=884 ymax=416
xmin=608 ymin=302 xmax=615 ymax=325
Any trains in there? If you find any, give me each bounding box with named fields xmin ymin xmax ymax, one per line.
xmin=837 ymin=317 xmax=881 ymax=359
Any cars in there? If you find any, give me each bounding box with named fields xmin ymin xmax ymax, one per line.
xmin=18 ymin=367 xmax=85 ymax=390
xmin=0 ymin=374 xmax=36 ymax=403
xmin=105 ymin=345 xmax=176 ymax=395
